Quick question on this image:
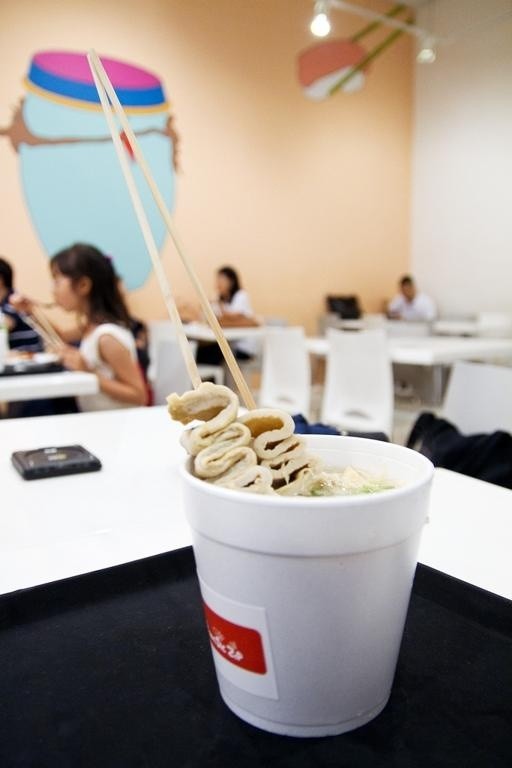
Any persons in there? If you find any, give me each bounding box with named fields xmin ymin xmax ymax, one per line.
xmin=388 ymin=275 xmax=443 ymax=329
xmin=11 ymin=237 xmax=154 ymax=414
xmin=128 ymin=314 xmax=156 ymax=407
xmin=0 ymin=254 xmax=45 ymax=359
xmin=196 ymin=264 xmax=266 ymax=369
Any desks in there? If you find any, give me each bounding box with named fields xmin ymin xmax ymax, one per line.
xmin=307 ymin=326 xmax=511 ymax=396
xmin=178 ymin=321 xmax=306 ymax=367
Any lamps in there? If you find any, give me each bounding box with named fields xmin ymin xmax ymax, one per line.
xmin=310 ymin=1 xmax=435 ymax=64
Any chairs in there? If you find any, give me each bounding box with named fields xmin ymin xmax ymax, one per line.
xmin=255 ymin=323 xmax=312 ymax=418
xmin=320 ymin=327 xmax=395 ymax=444
xmin=440 ymin=360 xmax=511 ymax=437
xmin=147 ymin=324 xmax=225 ymax=408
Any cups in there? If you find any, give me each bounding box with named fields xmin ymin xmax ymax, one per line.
xmin=182 ymin=435 xmax=436 ymax=740
